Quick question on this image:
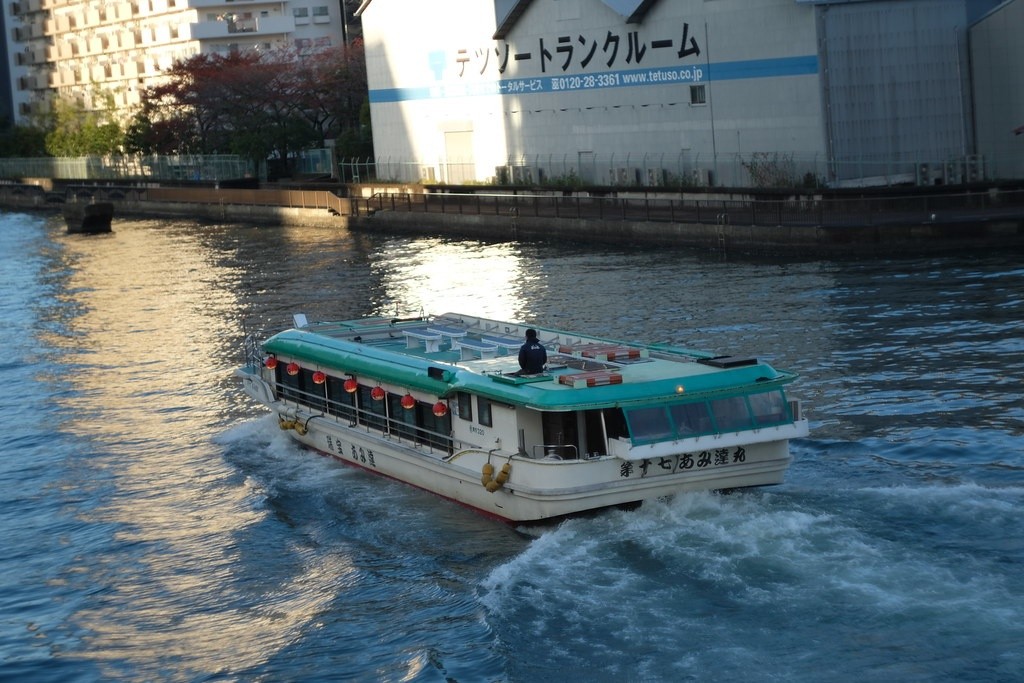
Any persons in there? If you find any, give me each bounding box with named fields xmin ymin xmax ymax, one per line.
xmin=518 ymin=329 xmax=548 ymax=374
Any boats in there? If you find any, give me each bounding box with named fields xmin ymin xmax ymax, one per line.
xmin=233 ymin=311 xmax=811 ymax=526
xmin=63 ymin=194 xmax=115 ymax=234
